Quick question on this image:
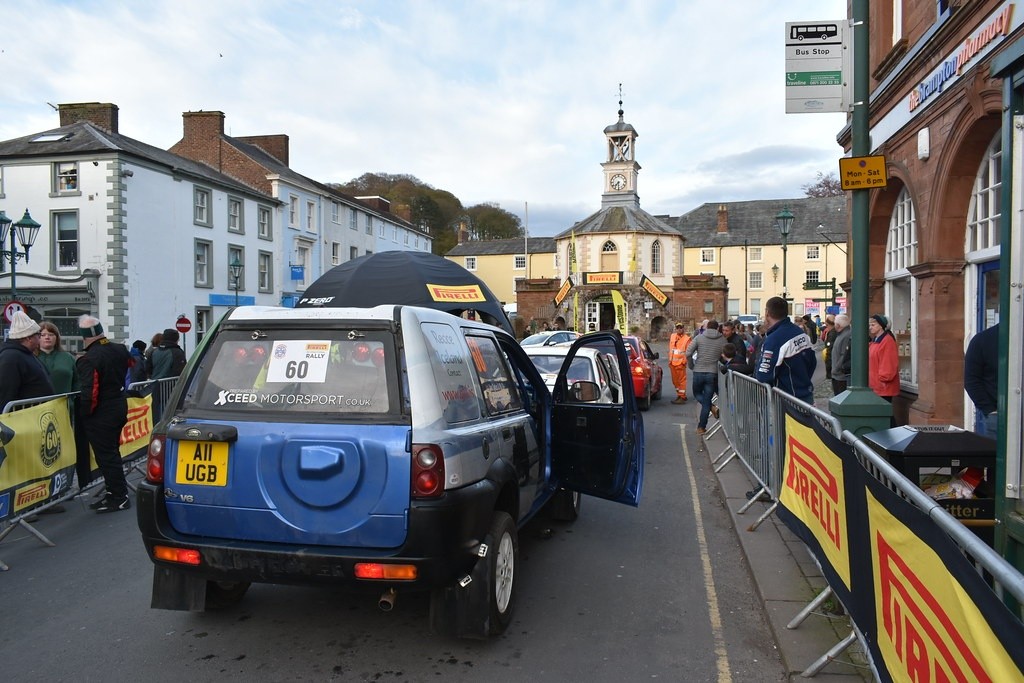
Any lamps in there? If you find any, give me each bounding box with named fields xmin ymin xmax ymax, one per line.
xmin=121 ymin=170 xmax=133 ymax=177
xmin=772 ymin=265 xmax=779 ymax=282
xmin=93 ymin=162 xmax=98 ymax=166
xmin=816 ymin=223 xmax=849 ymax=255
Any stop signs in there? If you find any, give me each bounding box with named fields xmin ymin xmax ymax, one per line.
xmin=176 ymin=318 xmax=191 ymax=333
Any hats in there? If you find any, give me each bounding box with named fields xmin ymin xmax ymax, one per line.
xmin=676 ymin=320 xmax=684 ymax=327
xmin=871 ymin=314 xmax=888 ymax=329
xmin=9 ymin=311 xmax=42 ymax=339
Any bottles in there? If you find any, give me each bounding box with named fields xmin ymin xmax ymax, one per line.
xmin=899 ymin=369 xmax=911 ymax=382
xmin=906 ymin=318 xmax=910 ymax=330
xmin=898 ymin=342 xmax=910 ymax=356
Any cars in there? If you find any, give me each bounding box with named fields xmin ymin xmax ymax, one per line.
xmin=737 ymin=314 xmax=759 ymax=327
xmin=493 ymin=346 xmax=624 ymax=404
xmin=519 ymin=331 xmax=583 ymax=366
xmin=584 ymin=336 xmax=663 ymax=411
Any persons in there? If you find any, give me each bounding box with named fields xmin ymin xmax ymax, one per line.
xmin=631 ymin=326 xmax=640 ymax=336
xmin=126 ymin=329 xmax=187 ymax=427
xmin=495 ymin=322 xmax=503 ymax=329
xmin=965 ymin=324 xmax=998 ymax=440
xmin=35 ymin=322 xmax=76 ymax=501
xmin=523 ymin=325 xmax=532 ymax=339
xmin=746 ymin=297 xmax=818 ymax=503
xmin=686 ymin=318 xmax=765 ymax=433
xmin=589 ymin=323 xmax=596 ymax=332
xmin=0 ymin=310 xmax=65 ymax=524
xmin=868 ymin=313 xmax=900 ymax=428
xmin=543 ymin=322 xmax=561 ymax=331
xmin=76 ymin=315 xmax=132 ymax=512
xmin=668 ymin=323 xmax=693 ymax=403
xmin=794 ymin=314 xmax=851 ymax=396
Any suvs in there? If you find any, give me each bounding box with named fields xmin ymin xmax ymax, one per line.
xmin=133 ymin=303 xmax=648 ymax=638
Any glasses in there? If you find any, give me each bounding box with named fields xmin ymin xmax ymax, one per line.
xmin=34 ymin=331 xmax=41 ymax=338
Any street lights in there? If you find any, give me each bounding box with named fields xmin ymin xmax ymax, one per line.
xmin=229 ymin=257 xmax=245 ymax=306
xmin=775 ymin=202 xmax=796 ymax=300
xmin=0 ymin=208 xmax=41 ymax=301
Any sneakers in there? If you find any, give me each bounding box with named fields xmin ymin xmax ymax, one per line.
xmin=97 ymin=494 xmax=130 ymax=513
xmin=90 ymin=491 xmax=112 ymax=508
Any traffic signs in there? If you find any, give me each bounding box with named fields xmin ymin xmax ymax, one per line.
xmin=803 ymin=286 xmax=833 ymax=290
xmin=802 ymin=281 xmax=833 ymax=286
xmin=813 ymin=299 xmax=833 ymax=302
xmin=825 ymin=306 xmax=839 ymax=314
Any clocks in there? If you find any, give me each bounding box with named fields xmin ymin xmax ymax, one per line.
xmin=610 ymin=174 xmax=627 ymax=190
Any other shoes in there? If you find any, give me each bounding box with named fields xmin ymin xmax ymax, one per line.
xmin=711 ymin=405 xmax=719 ymax=418
xmin=23 ymin=514 xmax=38 ymax=523
xmin=671 ymin=397 xmax=685 ymax=404
xmin=696 ymin=428 xmax=707 ymax=435
xmin=37 ymin=505 xmax=64 ymax=514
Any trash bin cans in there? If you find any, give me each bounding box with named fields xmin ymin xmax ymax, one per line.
xmin=864 ymin=423 xmax=995 ymax=578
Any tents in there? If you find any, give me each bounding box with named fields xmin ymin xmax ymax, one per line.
xmin=293 ymin=250 xmax=516 ymax=339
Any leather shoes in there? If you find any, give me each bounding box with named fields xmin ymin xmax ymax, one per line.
xmin=746 ymin=488 xmax=771 ymax=501
